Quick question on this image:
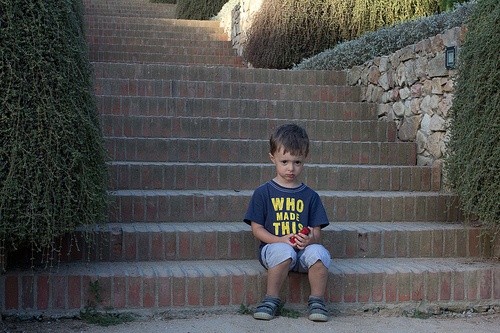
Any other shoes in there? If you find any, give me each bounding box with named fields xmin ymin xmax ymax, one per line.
xmin=308 ymin=298 xmax=328 ymax=322
xmin=254 ymin=296 xmax=280 ymax=319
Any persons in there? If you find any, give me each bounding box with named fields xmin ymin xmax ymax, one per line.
xmin=244 ymin=124 xmax=335 ymax=322
